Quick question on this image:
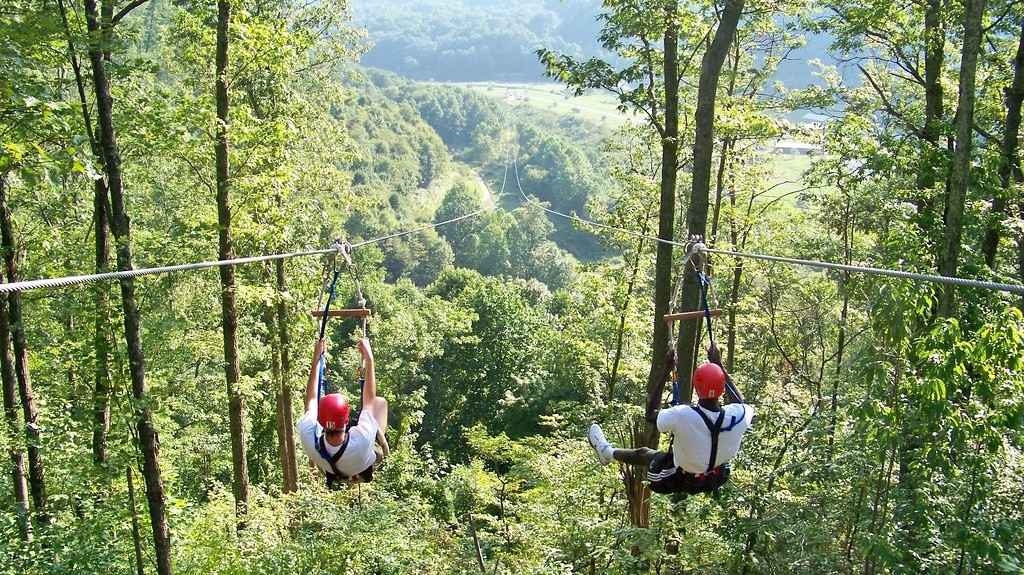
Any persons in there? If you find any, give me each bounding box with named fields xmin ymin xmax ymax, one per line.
xmin=296 ymin=338 xmax=390 ymax=485
xmin=587 ymin=346 xmax=754 ymax=495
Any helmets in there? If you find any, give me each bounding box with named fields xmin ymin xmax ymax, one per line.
xmin=693 ymin=363 xmax=726 ymax=399
xmin=317 ymin=393 xmax=349 ymax=430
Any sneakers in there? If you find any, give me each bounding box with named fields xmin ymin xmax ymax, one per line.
xmin=589 ymin=423 xmax=611 ymax=466
xmin=375 ymin=433 xmax=389 ymax=444
